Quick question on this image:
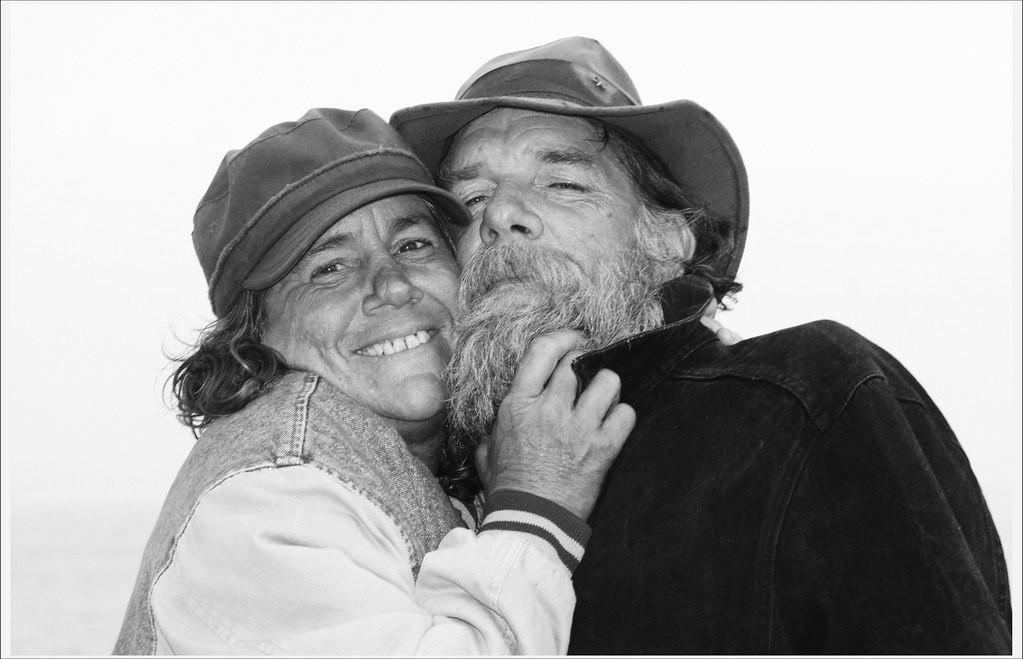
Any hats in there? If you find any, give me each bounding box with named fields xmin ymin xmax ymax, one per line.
xmin=389 ymin=37 xmax=750 ymax=302
xmin=192 ymin=108 xmax=474 ymax=322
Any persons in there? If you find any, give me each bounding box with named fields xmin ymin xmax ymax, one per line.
xmin=111 ymin=107 xmax=636 ymax=656
xmin=387 ymin=37 xmax=1012 ymax=655
